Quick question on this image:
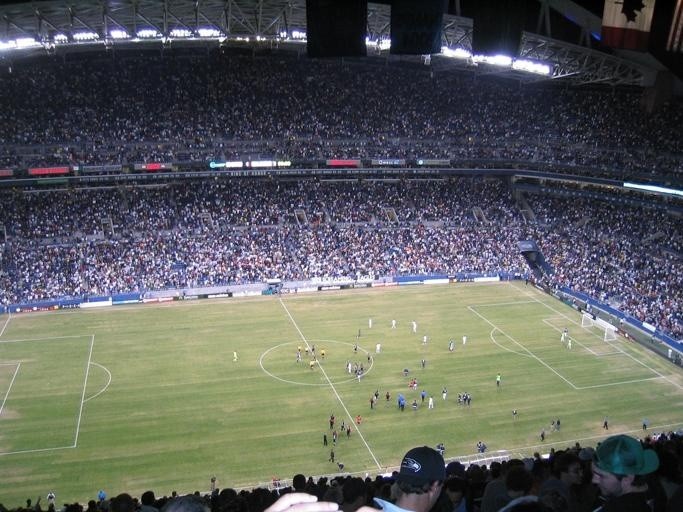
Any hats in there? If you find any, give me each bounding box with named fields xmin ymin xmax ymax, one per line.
xmin=399 ymin=446 xmax=447 ymax=481
xmin=592 ymin=435 xmax=660 ymax=476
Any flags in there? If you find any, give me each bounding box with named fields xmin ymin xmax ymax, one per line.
xmin=602 ymin=0 xmax=683 ymax=55
